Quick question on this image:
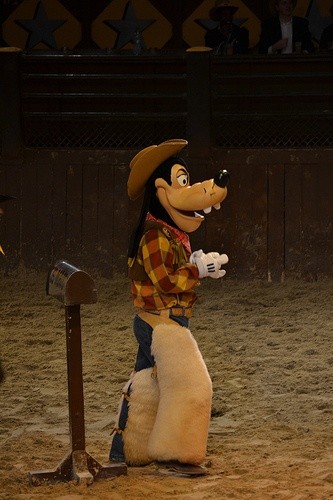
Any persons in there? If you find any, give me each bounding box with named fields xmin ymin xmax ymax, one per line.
xmin=204 ymin=0 xmax=249 ymax=56
xmin=259 ymin=0 xmax=333 ymax=54
xmin=108 ymin=137 xmax=232 ymax=475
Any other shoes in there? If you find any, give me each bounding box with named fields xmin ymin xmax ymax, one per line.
xmin=154 ymin=461 xmax=209 ymax=475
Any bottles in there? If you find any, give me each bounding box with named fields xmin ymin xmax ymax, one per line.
xmin=132 ymin=28 xmax=145 ymax=57
xmin=63 ymin=45 xmax=67 ymax=55
